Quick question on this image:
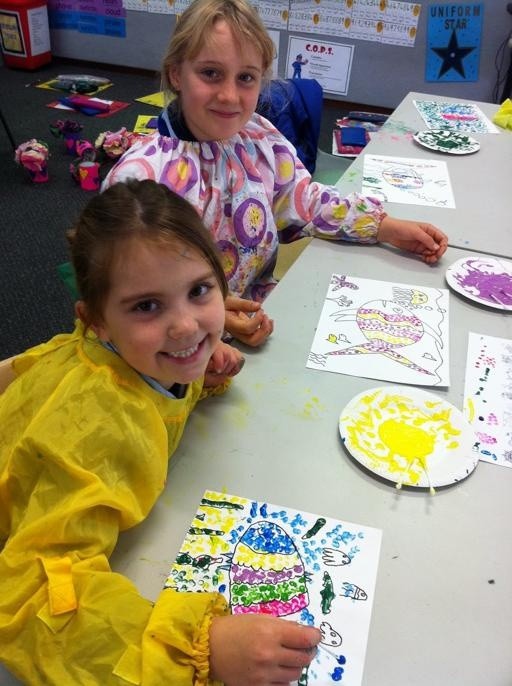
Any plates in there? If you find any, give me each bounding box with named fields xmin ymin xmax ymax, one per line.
xmin=445 ymin=256 xmax=511 ymax=312
xmin=336 ymin=385 xmax=480 ymax=488
xmin=413 ymin=127 xmax=480 ymax=154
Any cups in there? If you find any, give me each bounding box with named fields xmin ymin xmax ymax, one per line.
xmin=76 ymin=139 xmax=97 ymax=162
xmin=79 ymin=161 xmax=100 ymax=191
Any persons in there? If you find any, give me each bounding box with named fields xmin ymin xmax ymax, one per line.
xmin=98 ymin=0 xmax=448 ymax=348
xmin=291 ymin=53 xmax=308 ymax=79
xmin=0 ymin=177 xmax=322 ymax=685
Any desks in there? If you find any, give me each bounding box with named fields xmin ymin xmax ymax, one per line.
xmin=0 ymin=234 xmax=510 ymax=686
xmin=330 ymin=87 xmax=511 ymax=257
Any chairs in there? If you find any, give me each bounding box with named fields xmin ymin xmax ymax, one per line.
xmin=263 ymin=77 xmax=354 ymax=186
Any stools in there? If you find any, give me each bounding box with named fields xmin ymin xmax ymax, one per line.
xmin=272 ymin=235 xmax=315 ymax=280
